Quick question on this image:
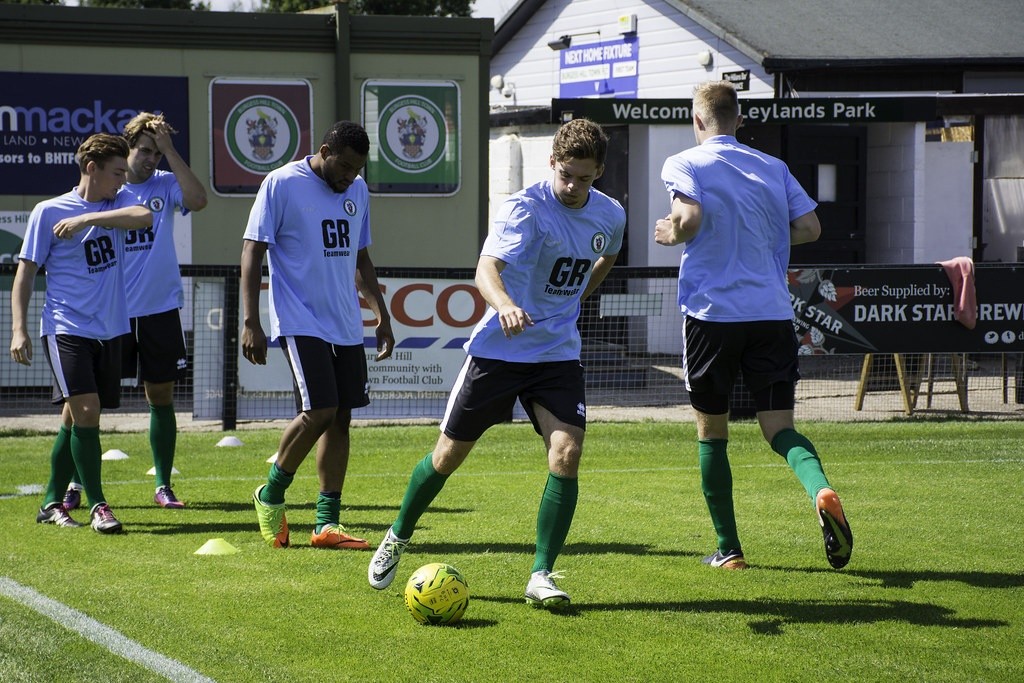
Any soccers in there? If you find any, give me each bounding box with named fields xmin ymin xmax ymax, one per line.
xmin=403 ymin=562 xmax=471 ymax=626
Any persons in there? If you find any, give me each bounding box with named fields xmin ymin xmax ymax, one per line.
xmin=368 ymin=119 xmax=626 ymax=609
xmin=240 ymin=120 xmax=395 ymax=551
xmin=654 ymin=80 xmax=853 ymax=571
xmin=10 ymin=134 xmax=154 ymax=534
xmin=62 ymin=112 xmax=209 ymax=510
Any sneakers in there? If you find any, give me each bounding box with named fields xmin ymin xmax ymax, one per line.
xmin=368 ymin=525 xmax=411 ymax=590
xmin=253 ymin=484 xmax=291 ymax=548
xmin=62 ymin=488 xmax=81 ymax=510
xmin=525 ymin=570 xmax=570 ymax=608
xmin=90 ymin=502 xmax=123 ymax=534
xmin=700 ymin=548 xmax=746 ymax=569
xmin=312 ymin=524 xmax=368 ymax=548
xmin=816 ymin=487 xmax=853 ymax=569
xmin=155 ymin=483 xmax=185 ymax=508
xmin=36 ymin=502 xmax=79 ymax=527
